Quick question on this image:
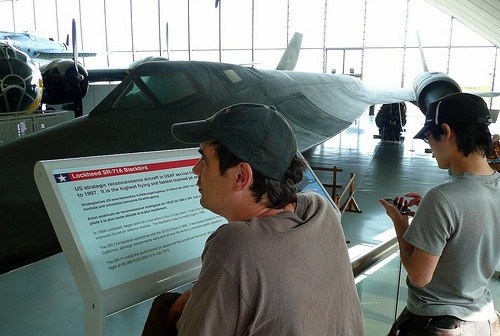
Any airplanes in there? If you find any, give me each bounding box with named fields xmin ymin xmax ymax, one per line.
xmin=0 ymin=0 xmax=500 ymax=277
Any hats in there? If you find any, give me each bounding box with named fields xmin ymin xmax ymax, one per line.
xmin=414 ymin=93 xmax=492 ymax=138
xmin=172 ymin=103 xmax=297 ymax=180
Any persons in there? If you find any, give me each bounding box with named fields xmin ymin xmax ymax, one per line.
xmin=142 ymin=102 xmax=366 ymax=336
xmin=379 ymin=92 xmax=500 ymax=336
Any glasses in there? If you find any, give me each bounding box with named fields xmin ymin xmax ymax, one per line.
xmin=422 ymin=133 xmax=431 ymax=143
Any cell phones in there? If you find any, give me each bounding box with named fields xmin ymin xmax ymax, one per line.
xmin=379 ymin=198 xmax=411 ymax=214
